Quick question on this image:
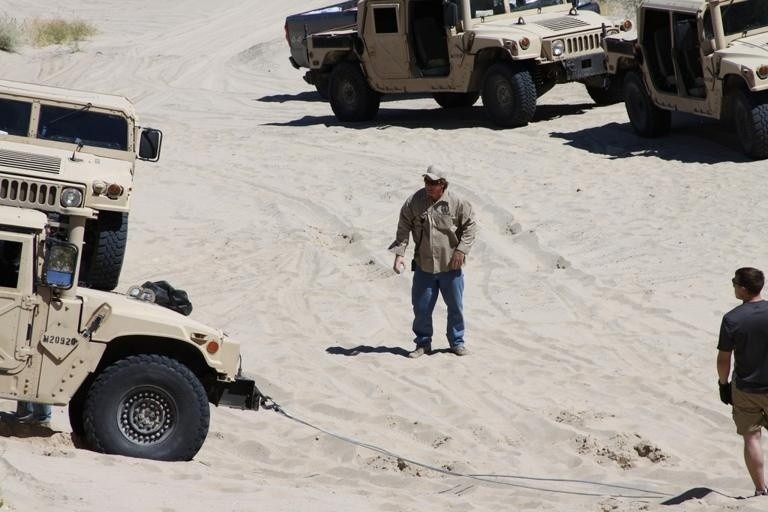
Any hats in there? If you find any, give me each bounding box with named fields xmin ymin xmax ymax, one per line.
xmin=422 ymin=163 xmax=447 ymax=180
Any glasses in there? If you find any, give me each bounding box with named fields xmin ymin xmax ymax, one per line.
xmin=424 ymin=177 xmax=440 ymax=185
xmin=732 ymin=278 xmax=744 ymax=286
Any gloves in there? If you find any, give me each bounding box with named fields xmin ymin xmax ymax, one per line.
xmin=718 ymin=380 xmax=732 ymax=404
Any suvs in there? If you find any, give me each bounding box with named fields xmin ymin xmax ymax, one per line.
xmin=1 ymin=77 xmax=166 ymax=291
xmin=303 ymin=0 xmax=633 ymax=131
xmin=598 ymin=0 xmax=767 ymax=161
xmin=1 ymin=203 xmax=269 ymax=466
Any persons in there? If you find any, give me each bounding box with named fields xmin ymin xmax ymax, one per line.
xmin=387 ymin=165 xmax=479 ymax=359
xmin=11 ymin=400 xmax=51 ymax=429
xmin=714 ymin=266 xmax=768 ymax=494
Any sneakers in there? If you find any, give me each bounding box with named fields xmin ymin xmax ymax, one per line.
xmin=451 ymin=346 xmax=466 ymax=356
xmin=408 ymin=344 xmax=431 ymax=358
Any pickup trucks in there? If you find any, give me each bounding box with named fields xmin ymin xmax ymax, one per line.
xmin=281 ymin=1 xmax=359 ymax=89
